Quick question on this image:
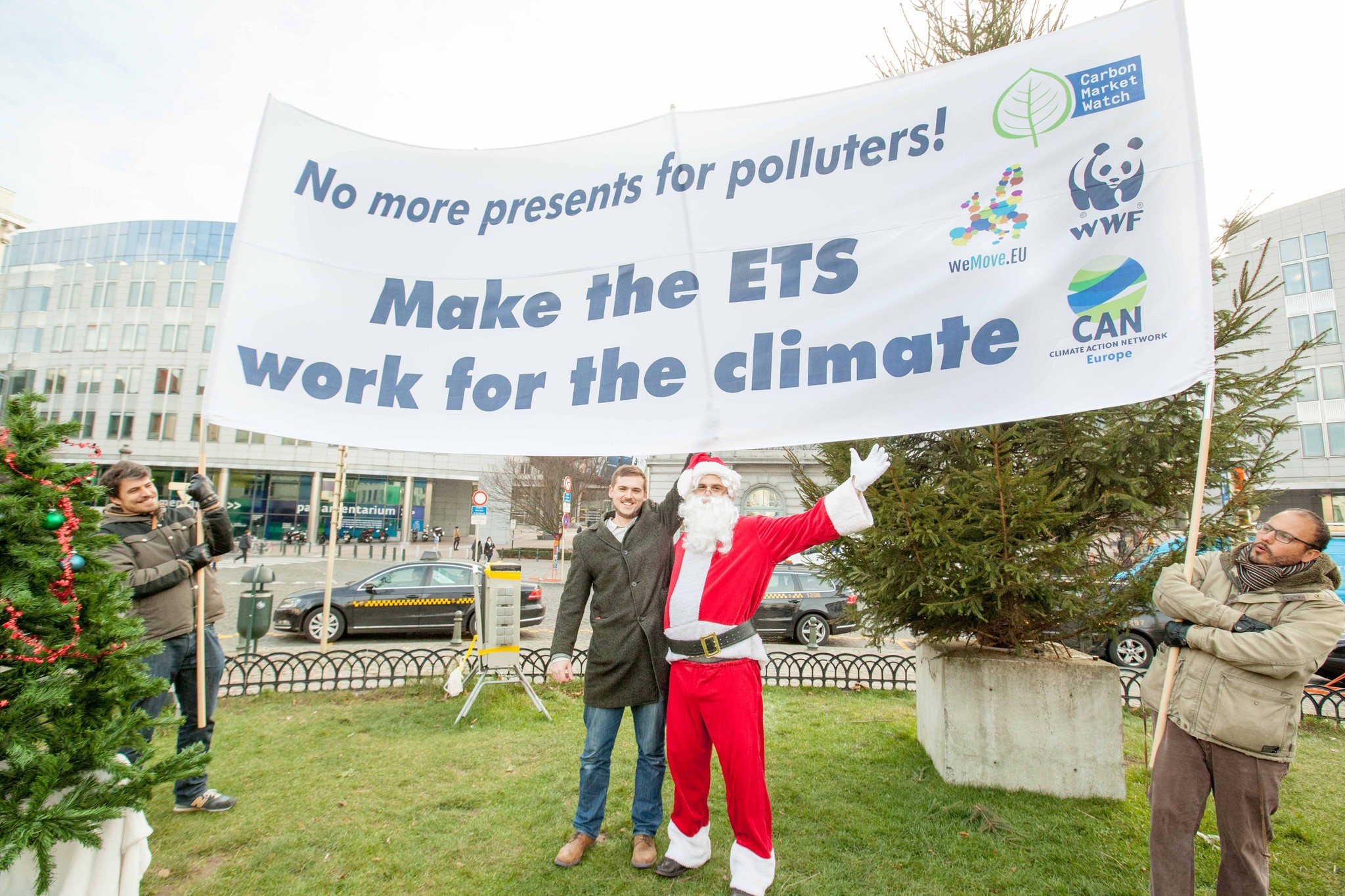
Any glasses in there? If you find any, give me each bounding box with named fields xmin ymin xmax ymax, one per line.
xmin=692 ymin=486 xmax=728 ymax=498
xmin=1256 ymin=520 xmax=1323 ymax=551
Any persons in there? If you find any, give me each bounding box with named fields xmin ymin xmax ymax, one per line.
xmin=453 ymin=526 xmax=460 ymax=550
xmin=234 ymin=530 xmax=251 ymax=563
xmin=211 ymin=555 xmax=221 ymax=572
xmin=89 ymin=461 xmax=237 ymax=813
xmin=1142 ymin=508 xmax=1345 ymax=896
xmin=484 ymin=537 xmax=495 ymax=562
xmin=587 ymin=519 xmax=592 ymax=527
xmin=656 ymin=443 xmax=890 ymax=896
xmin=551 ymin=452 xmax=714 ymax=867
xmin=578 ymin=525 xmax=582 ymax=532
xmin=471 ymin=537 xmax=483 ymax=562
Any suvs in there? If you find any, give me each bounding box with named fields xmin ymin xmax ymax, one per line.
xmin=752 ymin=564 xmax=861 ymax=647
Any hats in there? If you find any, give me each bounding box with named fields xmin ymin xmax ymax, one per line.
xmin=676 ymin=452 xmax=741 ymax=498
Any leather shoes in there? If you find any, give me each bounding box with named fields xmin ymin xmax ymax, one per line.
xmin=555 ymin=830 xmax=596 ymax=866
xmin=630 ymin=833 xmax=658 ymax=868
xmin=654 ymin=856 xmax=688 ymax=877
xmin=729 ymin=887 xmax=755 ymax=896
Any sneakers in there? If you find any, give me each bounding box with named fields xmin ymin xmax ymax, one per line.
xmin=172 ymin=787 xmax=236 ymax=811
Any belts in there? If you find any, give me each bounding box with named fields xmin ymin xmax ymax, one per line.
xmin=667 ymin=617 xmax=756 ymax=658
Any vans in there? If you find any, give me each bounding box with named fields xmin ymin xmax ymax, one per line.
xmin=1113 ymin=535 xmax=1345 ymax=602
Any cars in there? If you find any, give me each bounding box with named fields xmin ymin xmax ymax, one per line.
xmin=273 ymin=550 xmax=546 ymax=643
xmin=1059 ymin=596 xmax=1345 ymax=683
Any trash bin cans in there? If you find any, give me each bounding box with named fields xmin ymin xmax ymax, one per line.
xmin=236 ymin=563 xmax=275 ymax=639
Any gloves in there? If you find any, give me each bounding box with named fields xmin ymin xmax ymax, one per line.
xmin=1232 ymin=615 xmax=1274 ymax=634
xmin=1163 ymin=620 xmax=1197 ymax=648
xmin=185 ymin=474 xmax=221 ymax=510
xmin=177 ymin=543 xmax=212 ymax=575
xmin=849 ymin=443 xmax=891 ymax=492
